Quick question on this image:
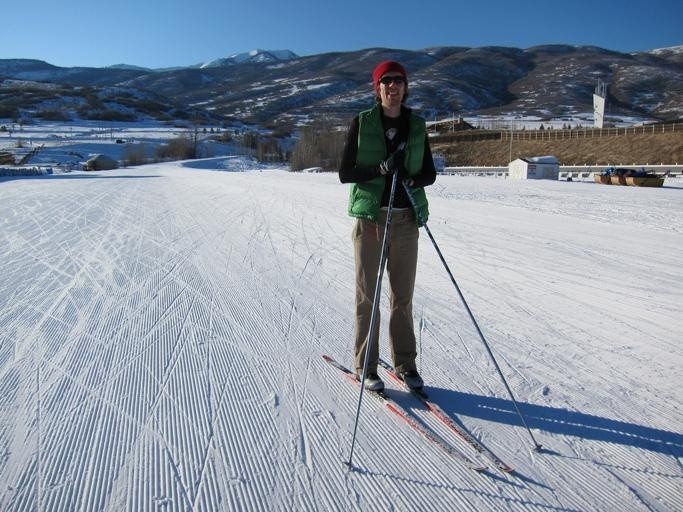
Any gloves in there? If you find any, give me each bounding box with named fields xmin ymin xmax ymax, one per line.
xmin=394 ymin=148 xmax=413 ymax=187
xmin=381 ymin=143 xmax=406 ymax=174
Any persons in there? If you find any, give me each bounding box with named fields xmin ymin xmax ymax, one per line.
xmin=341 ymin=61 xmax=436 ymax=390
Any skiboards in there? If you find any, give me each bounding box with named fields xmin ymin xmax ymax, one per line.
xmin=320 ymin=353 xmax=517 ymax=474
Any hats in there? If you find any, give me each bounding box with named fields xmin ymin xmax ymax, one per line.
xmin=373 ymin=60 xmax=407 ymax=94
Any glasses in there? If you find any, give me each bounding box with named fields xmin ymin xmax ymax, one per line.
xmin=379 ymin=76 xmax=405 ymax=84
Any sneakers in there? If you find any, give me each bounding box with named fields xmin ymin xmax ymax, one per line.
xmin=359 ymin=372 xmax=386 ymax=391
xmin=394 ymin=368 xmax=424 ymax=388
